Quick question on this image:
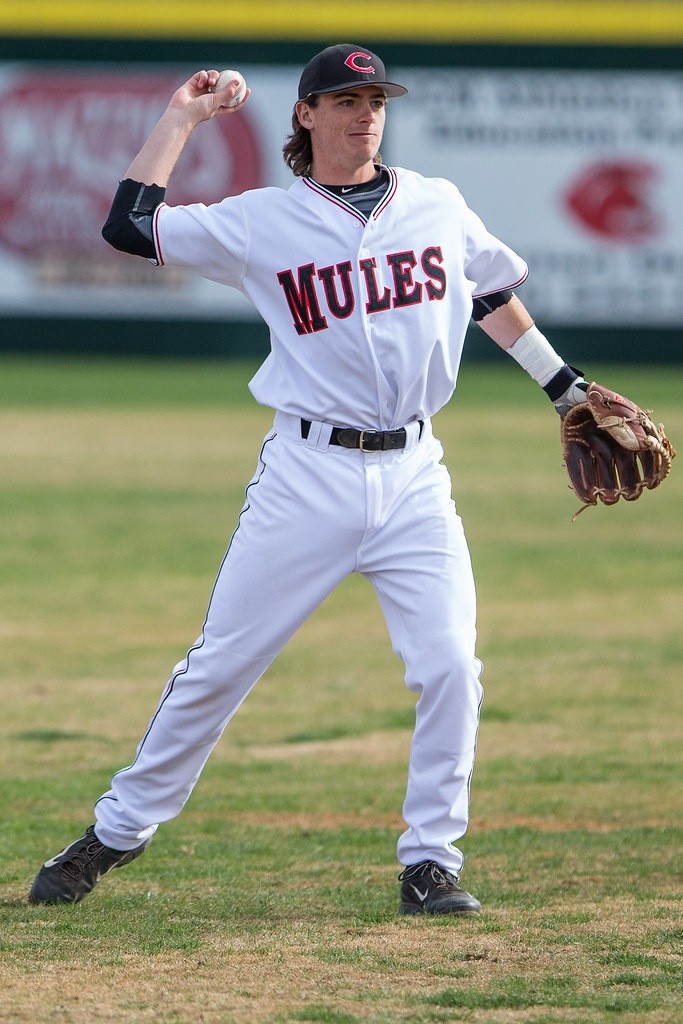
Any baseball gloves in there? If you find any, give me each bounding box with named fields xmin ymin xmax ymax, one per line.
xmin=560 ymin=380 xmax=677 ymax=507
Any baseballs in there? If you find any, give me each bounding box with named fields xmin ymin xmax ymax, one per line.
xmin=211 ymin=69 xmax=247 ymax=109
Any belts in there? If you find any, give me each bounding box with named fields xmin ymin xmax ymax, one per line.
xmin=299 ymin=418 xmax=424 ymax=453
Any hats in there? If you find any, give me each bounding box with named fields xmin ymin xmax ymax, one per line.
xmin=298 ymin=45 xmax=408 ymax=102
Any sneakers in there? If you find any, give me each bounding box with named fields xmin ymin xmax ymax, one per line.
xmin=398 ymin=861 xmax=481 ymax=917
xmin=29 ymin=825 xmax=153 ymax=906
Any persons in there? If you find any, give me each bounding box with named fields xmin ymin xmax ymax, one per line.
xmin=29 ymin=43 xmax=677 ymax=915
